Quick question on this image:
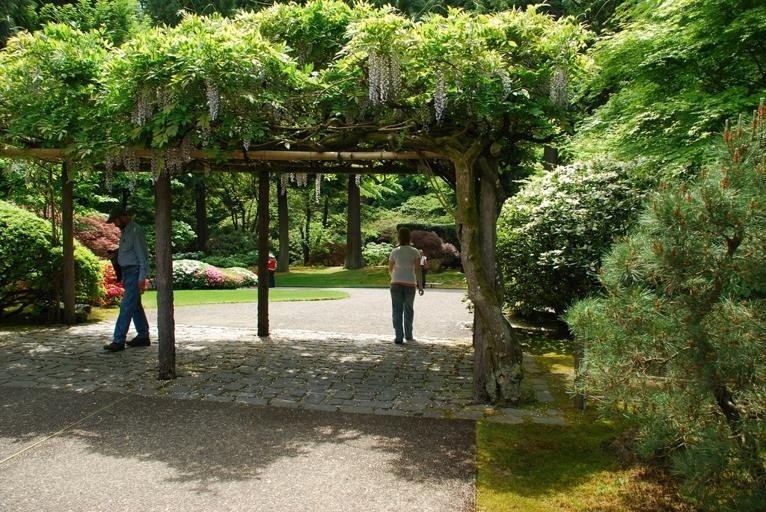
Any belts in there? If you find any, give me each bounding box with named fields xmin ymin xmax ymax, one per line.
xmin=121 ymin=265 xmax=138 ymax=270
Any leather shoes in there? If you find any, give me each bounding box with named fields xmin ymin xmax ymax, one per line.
xmin=104 ymin=341 xmax=125 ymax=352
xmin=126 ymin=337 xmax=150 ymax=346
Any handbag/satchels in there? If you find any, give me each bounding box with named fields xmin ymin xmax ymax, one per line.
xmin=110 ymin=252 xmax=123 ymax=284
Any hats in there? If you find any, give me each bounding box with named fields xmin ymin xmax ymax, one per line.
xmin=105 ymin=207 xmax=127 ymax=224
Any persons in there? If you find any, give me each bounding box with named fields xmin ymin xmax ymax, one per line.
xmin=417 ymin=249 xmax=427 ymax=288
xmin=103 ymin=204 xmax=152 ymax=351
xmin=267 ymin=250 xmax=277 ymax=288
xmin=388 ymin=226 xmax=424 ymax=345
